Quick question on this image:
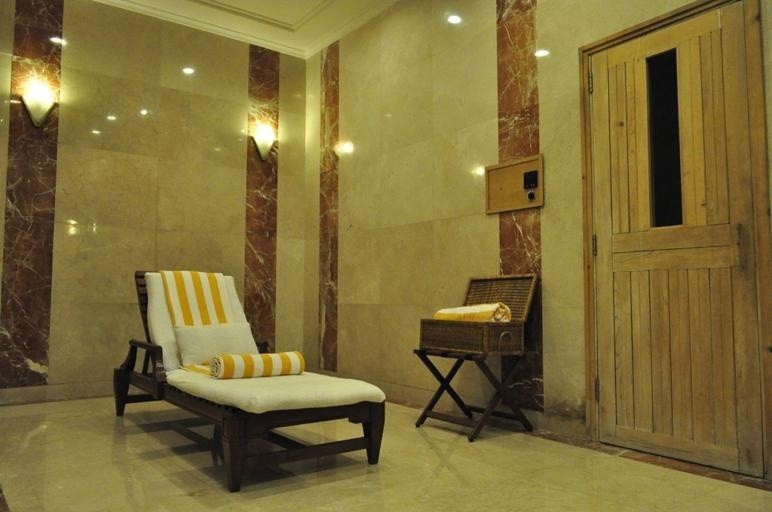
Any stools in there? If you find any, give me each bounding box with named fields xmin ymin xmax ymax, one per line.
xmin=412 ymin=349 xmax=533 ymax=440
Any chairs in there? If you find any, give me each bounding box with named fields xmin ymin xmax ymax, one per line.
xmin=115 ymin=270 xmax=387 ymax=492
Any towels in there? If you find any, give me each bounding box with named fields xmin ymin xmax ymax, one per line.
xmin=179 ymin=350 xmax=305 ymax=378
xmin=432 ymin=302 xmax=511 ymax=323
